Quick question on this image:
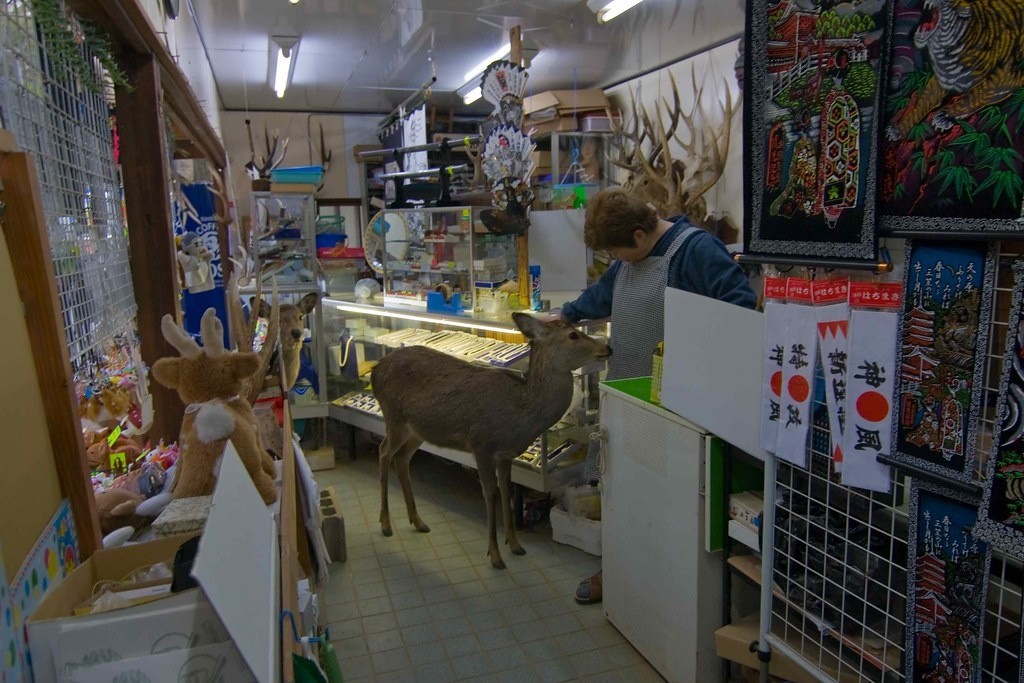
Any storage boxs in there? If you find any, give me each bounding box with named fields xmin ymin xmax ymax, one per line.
xmin=727 ymin=492 xmax=764 ymax=553
xmin=715 ymin=607 xmax=876 ymax=683
xmin=550 ymin=505 xmax=601 ymax=556
xmin=24 ymin=438 xmax=284 ymax=683
xmin=271 ymin=165 xmax=324 ymax=184
xmin=520 ymin=90 xmax=622 ymax=211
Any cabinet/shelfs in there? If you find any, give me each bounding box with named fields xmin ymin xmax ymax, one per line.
xmin=0 ymin=0 xmax=335 ymax=682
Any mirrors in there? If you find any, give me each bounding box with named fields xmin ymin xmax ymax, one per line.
xmin=363 ymin=211 xmax=410 ymax=293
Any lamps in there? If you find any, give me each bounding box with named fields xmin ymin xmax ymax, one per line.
xmin=587 ymin=0 xmax=643 ymax=24
xmin=266 ymin=35 xmax=301 ymax=98
xmin=454 ymin=38 xmax=540 ymax=106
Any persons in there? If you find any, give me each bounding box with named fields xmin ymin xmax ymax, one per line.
xmin=534 ymin=184 xmax=757 ymax=605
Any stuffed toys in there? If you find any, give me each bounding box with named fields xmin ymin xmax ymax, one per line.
xmin=81 ymin=308 xmax=284 ymax=551
xmin=175 ymin=233 xmax=217 ymax=295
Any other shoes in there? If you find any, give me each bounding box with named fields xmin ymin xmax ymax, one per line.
xmin=573 ymin=568 xmax=602 ymax=604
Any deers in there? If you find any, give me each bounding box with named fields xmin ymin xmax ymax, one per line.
xmin=592 ymin=65 xmax=734 ymax=222
xmin=366 ymin=313 xmax=614 ymax=571
xmin=231 ymin=291 xmax=319 ymax=396
